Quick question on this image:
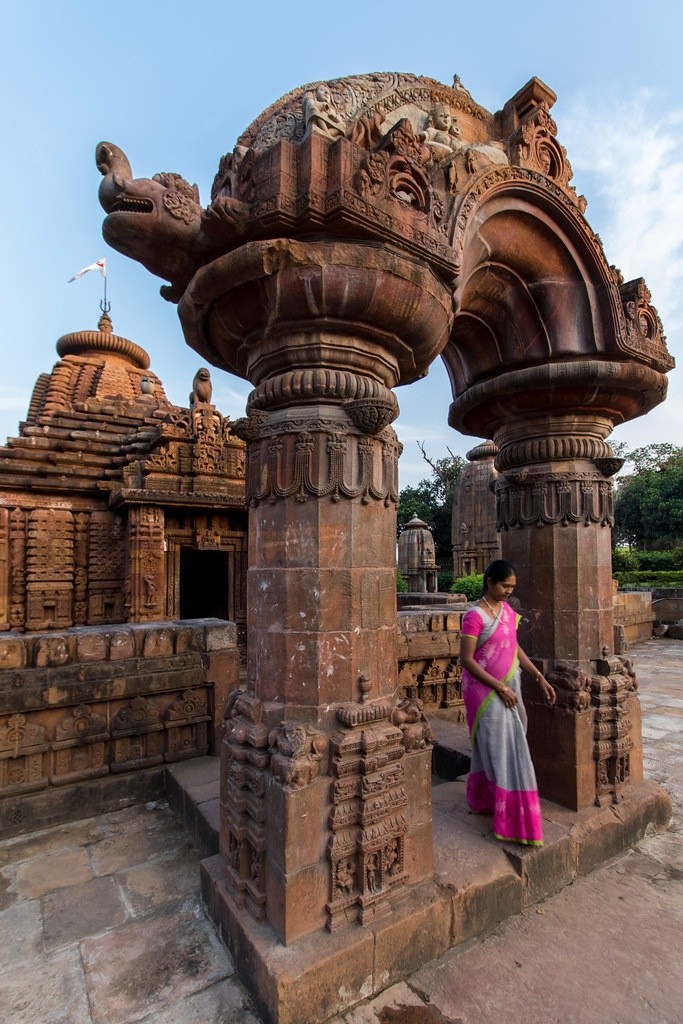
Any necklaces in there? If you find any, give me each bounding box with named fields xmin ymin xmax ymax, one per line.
xmin=482 ymin=596 xmax=507 ymax=625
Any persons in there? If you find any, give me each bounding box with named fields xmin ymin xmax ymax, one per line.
xmin=460 ymin=561 xmax=555 ymax=846
xmin=144 ymin=574 xmax=157 ymax=603
xmin=427 ymin=101 xmax=463 ymax=149
xmin=368 ymin=856 xmax=379 ymax=893
xmin=125 ymin=575 xmax=131 ymax=604
xmin=75 ymin=571 xmax=87 ymax=601
xmin=639 ymin=315 xmax=648 ymax=336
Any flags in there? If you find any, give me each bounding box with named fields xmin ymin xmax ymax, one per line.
xmin=70 ymin=258 xmax=106 ymax=281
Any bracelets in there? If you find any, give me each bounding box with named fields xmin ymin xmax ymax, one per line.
xmin=499 ymin=684 xmax=507 ymax=693
xmin=536 ymin=672 xmax=540 ymax=682
